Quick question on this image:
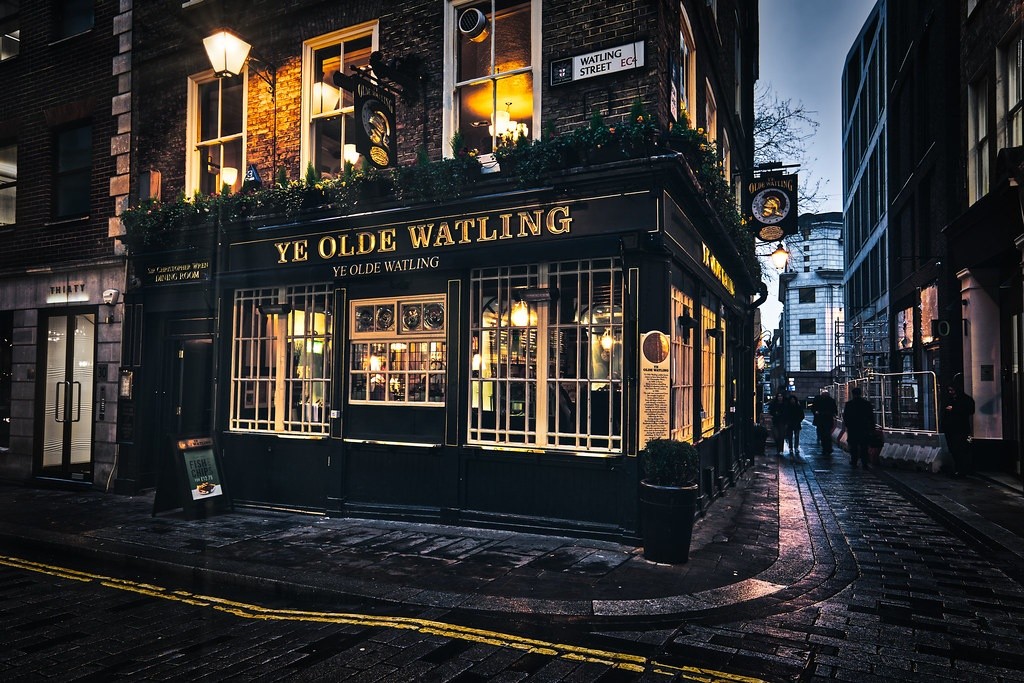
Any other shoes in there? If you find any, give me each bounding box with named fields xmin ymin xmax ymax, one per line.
xmin=818 ymin=440 xmax=821 ymax=445
xmin=776 ymin=452 xmax=779 ymax=455
xmin=861 ymin=456 xmax=871 ymax=462
xmin=822 ymin=448 xmax=828 ymax=453
xmin=789 ymin=450 xmax=793 ymax=453
xmin=826 ymin=449 xmax=834 ymax=456
xmin=953 ymin=472 xmax=960 ymax=475
xmin=780 ymin=452 xmax=783 ymax=456
xmin=850 ymin=461 xmax=857 ymax=466
xmin=795 ymin=449 xmax=799 ymax=453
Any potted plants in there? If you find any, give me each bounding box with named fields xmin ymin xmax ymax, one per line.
xmin=637 ymin=437 xmax=700 ymax=565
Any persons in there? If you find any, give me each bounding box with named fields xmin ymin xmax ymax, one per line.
xmin=783 ymin=394 xmax=804 ymax=454
xmin=810 ymin=390 xmax=838 ymax=457
xmin=843 ymin=387 xmax=875 ymax=469
xmin=940 ymin=381 xmax=975 ymax=479
xmin=769 ymin=393 xmax=786 ymax=455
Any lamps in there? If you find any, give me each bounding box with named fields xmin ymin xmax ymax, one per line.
xmin=204 ymin=20 xmax=277 ymax=103
